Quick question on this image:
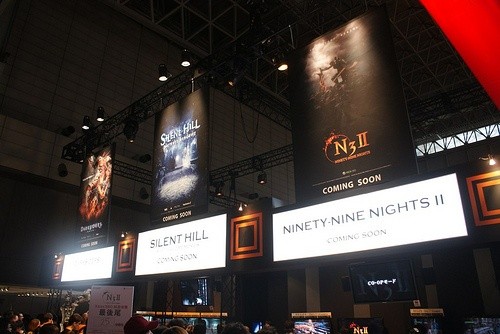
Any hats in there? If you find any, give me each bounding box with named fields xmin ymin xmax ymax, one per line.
xmin=174 ymin=318 xmax=187 ymax=326
xmin=124 ymin=316 xmax=158 ymax=334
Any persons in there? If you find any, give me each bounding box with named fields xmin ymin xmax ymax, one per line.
xmin=284 ymin=313 xmax=295 ymax=334
xmin=124 ymin=316 xmax=206 ymax=334
xmin=217 ymin=319 xmax=250 ymax=334
xmin=256 ymin=319 xmax=277 ymax=334
xmin=0 ymin=312 xmax=89 ymax=334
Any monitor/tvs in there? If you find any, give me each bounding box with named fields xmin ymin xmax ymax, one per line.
xmin=412 ymin=316 xmax=441 ymax=334
xmin=464 ymin=317 xmax=500 ymax=334
xmin=293 ymin=318 xmax=334 ymax=333
xmin=181 ymin=276 xmax=208 ymax=306
